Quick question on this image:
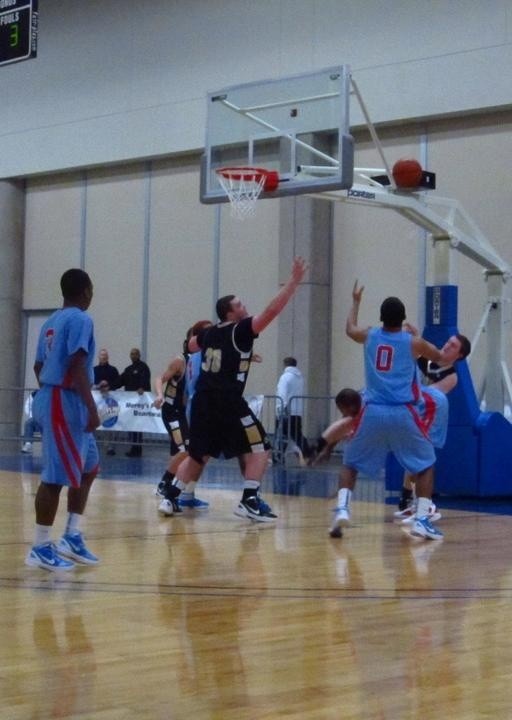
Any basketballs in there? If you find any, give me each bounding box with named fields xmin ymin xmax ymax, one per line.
xmin=393 ymin=158 xmax=422 ymax=188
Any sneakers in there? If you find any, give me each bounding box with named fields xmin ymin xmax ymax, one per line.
xmin=156 ymin=481 xmax=444 ymax=541
xmin=26 ymin=541 xmax=73 ymax=571
xmin=57 ymin=532 xmax=99 ymax=565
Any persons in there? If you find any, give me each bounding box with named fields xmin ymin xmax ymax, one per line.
xmin=31 ymin=268 xmax=100 ymax=572
xmin=93 ymin=347 xmax=151 ymax=457
xmin=154 ymin=256 xmax=306 ymax=522
xmin=22 ymin=390 xmax=41 ymax=453
xmin=270 ymin=356 xmax=312 ymax=466
xmin=309 ymin=280 xmax=471 ymax=541
